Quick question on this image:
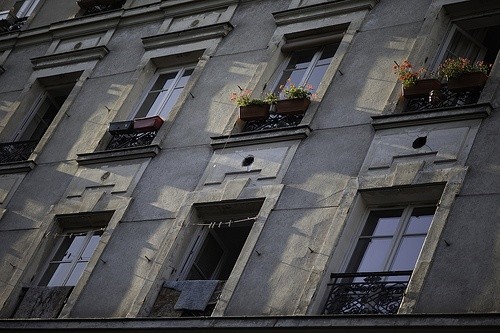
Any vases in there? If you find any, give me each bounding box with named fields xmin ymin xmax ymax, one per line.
xmin=446 ymin=72 xmax=487 ymax=90
xmin=401 ymin=78 xmax=442 ymax=97
xmin=108 ymin=119 xmax=134 ymax=136
xmin=275 ymin=97 xmax=310 ymax=114
xmin=238 ymin=101 xmax=273 ymax=120
xmin=133 ymin=114 xmax=164 ymax=133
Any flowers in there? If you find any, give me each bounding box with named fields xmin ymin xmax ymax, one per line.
xmin=439 ymin=55 xmax=491 ymax=79
xmin=230 ymin=89 xmax=258 ymax=105
xmin=281 ymin=77 xmax=316 ymax=99
xmin=391 ymin=59 xmax=426 ymax=85
